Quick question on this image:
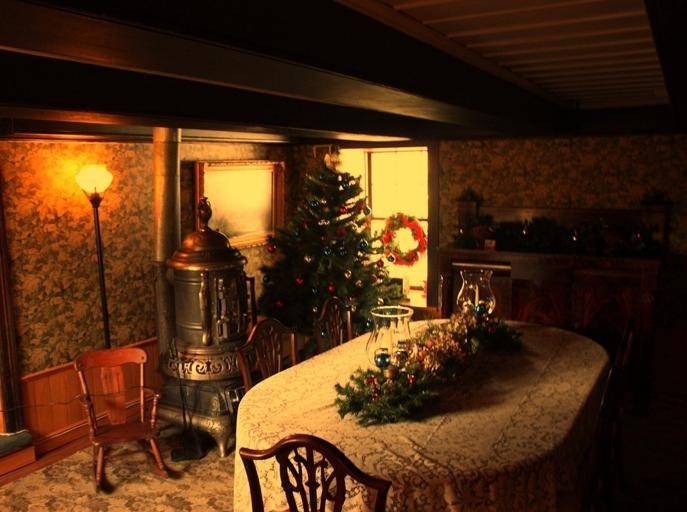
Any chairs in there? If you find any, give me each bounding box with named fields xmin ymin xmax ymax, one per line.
xmin=239 ymin=433 xmax=393 ymax=512
xmin=314 ymin=297 xmax=354 ymax=353
xmin=73 ymin=346 xmax=172 ymax=493
xmin=619 ymin=330 xmax=635 ymax=404
xmin=558 ymin=363 xmax=615 ymax=511
xmin=232 ymin=315 xmax=284 ymax=392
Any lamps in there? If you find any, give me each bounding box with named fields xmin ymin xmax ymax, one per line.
xmin=73 ymin=162 xmax=113 ymax=349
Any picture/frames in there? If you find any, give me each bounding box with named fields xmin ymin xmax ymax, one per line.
xmin=192 ymin=159 xmax=287 ymax=251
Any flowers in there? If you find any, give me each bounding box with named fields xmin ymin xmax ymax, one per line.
xmin=383 ymin=211 xmax=427 ymax=267
